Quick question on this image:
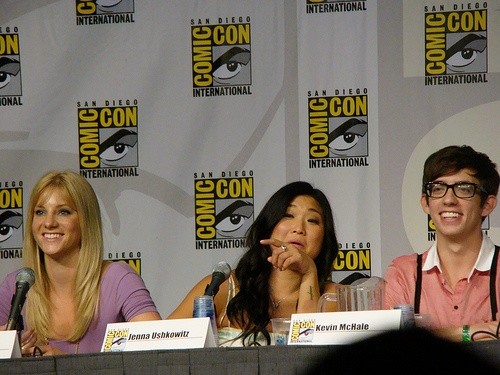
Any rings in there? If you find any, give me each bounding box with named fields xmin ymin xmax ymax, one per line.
xmin=281 ymin=245 xmax=287 ymax=252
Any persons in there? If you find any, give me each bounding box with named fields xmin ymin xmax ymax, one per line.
xmin=0 ymin=170 xmax=163 ymax=359
xmin=383 ymin=145 xmax=500 ymax=342
xmin=165 ymin=180 xmax=354 ymax=335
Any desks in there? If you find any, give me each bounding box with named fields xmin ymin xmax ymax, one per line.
xmin=0 ymin=344 xmax=348 ymax=375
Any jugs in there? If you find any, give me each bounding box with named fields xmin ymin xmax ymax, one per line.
xmin=317 ymin=275 xmax=386 ymax=313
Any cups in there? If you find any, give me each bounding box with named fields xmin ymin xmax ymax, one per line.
xmin=269 ymin=316 xmax=293 ymax=346
xmin=192 ymin=295 xmax=218 ymax=348
xmin=392 ymin=305 xmax=417 ymax=333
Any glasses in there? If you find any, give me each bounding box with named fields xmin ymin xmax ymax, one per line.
xmin=423 ymin=181 xmax=489 ymax=199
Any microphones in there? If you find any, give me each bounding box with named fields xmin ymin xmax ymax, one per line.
xmin=6 ymin=267 xmax=35 ymax=331
xmin=205 ymin=262 xmax=232 ymax=298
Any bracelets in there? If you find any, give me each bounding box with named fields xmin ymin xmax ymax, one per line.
xmin=462 ymin=325 xmax=470 ymax=341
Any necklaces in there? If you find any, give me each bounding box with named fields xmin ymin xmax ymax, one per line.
xmin=271 ymin=295 xmax=288 ymax=308
